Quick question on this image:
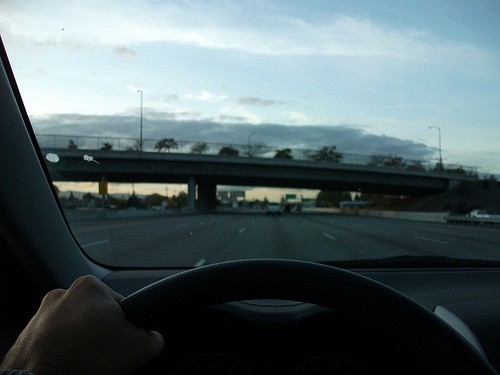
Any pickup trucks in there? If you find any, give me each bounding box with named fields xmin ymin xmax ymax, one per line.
xmin=466 ymin=209 xmax=500 ymax=218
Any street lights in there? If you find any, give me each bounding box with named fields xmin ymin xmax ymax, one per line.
xmin=248 ymin=132 xmax=256 ymax=159
xmin=429 ymin=126 xmax=442 ymax=174
xmin=138 ymin=90 xmax=143 ymax=151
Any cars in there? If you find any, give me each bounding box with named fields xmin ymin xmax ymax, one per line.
xmin=106 ymin=205 xmax=161 ymax=210
xmin=264 ymin=202 xmax=282 ymax=215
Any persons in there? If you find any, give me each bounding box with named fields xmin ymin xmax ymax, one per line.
xmin=0 ymin=272 xmax=165 ymax=375
